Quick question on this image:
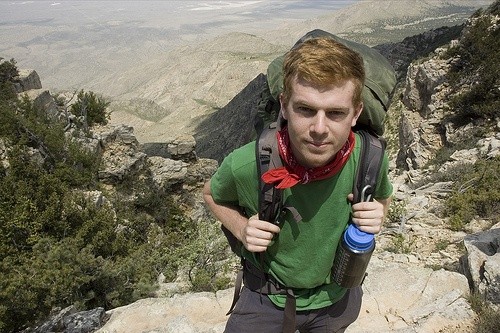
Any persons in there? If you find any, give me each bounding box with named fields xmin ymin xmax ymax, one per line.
xmin=201 ymin=35 xmax=394 ymax=333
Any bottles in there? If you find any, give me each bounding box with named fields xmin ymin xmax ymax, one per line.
xmin=332 ymin=222 xmax=376 ymax=288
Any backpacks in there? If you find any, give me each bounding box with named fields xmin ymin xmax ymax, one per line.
xmin=247 ymin=28 xmax=398 ymax=287
